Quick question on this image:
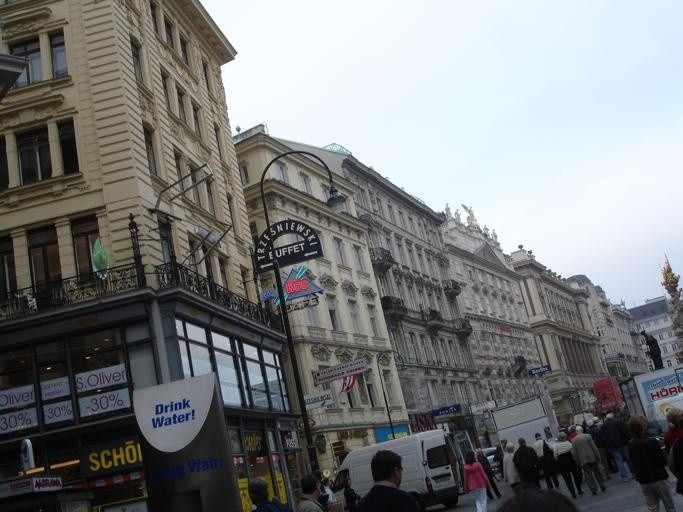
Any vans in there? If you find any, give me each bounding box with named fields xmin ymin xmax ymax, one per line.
xmin=574 ymin=413 xmax=595 ymax=426
xmin=326 ymin=428 xmax=462 ymax=509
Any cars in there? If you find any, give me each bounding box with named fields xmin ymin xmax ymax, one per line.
xmin=481 ymin=446 xmax=500 ymax=472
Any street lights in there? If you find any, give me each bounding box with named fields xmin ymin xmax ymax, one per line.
xmin=377 ymin=349 xmax=408 ymax=440
xmin=574 ymin=393 xmax=588 ymax=426
xmin=260 ymin=150 xmax=349 ymax=505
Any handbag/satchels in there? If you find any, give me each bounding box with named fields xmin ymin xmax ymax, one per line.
xmin=542 ymin=440 xmax=556 ymax=463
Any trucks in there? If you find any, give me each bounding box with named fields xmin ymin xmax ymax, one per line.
xmin=487 ymin=394 xmax=559 ymax=458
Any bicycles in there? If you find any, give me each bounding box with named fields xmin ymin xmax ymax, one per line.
xmin=493 ymin=465 xmax=504 ymax=482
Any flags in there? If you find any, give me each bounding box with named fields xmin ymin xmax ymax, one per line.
xmin=341 ymin=373 xmax=359 ymax=392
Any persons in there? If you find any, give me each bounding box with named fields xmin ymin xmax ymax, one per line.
xmin=294 ymin=474 xmax=327 ymax=512
xmin=461 ymin=450 xmax=492 ymax=512
xmin=640 ymin=329 xmax=664 ymax=370
xmin=246 ymin=476 xmax=290 ymax=512
xmin=499 ymin=411 xmax=634 ymax=497
xmin=662 ymin=407 xmax=681 ymax=454
xmin=444 ymin=202 xmax=498 ymax=242
xmin=321 ymin=477 xmax=336 ymax=502
xmin=475 ymin=447 xmax=504 ymax=500
xmin=668 ymin=411 xmax=683 ymax=496
xmin=356 ymin=448 xmax=418 ymax=511
xmin=624 ymin=413 xmax=676 ymax=511
xmin=342 ymin=478 xmax=360 ymax=512
xmin=494 ymin=486 xmax=581 ymax=512
